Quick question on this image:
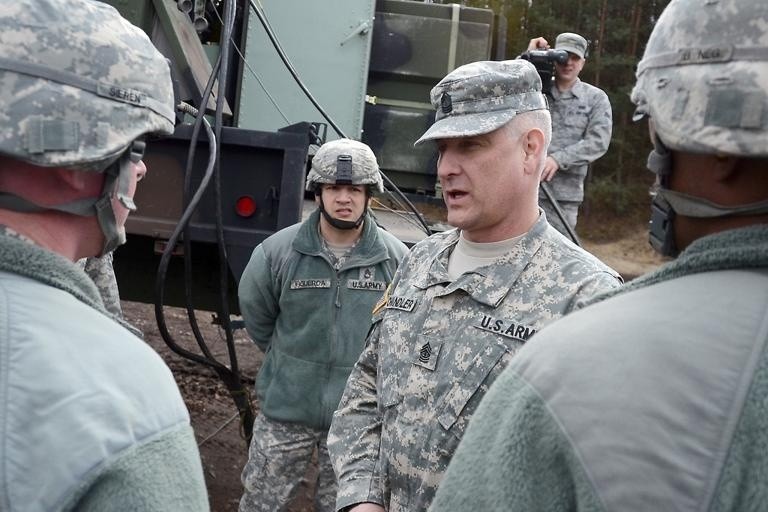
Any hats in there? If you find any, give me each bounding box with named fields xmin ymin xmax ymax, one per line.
xmin=414 ymin=60 xmax=551 ymax=148
xmin=555 ymin=32 xmax=588 ymax=61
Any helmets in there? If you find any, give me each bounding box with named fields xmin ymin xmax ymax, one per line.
xmin=1 ymin=0 xmax=177 ymax=172
xmin=630 ymin=0 xmax=768 ymax=159
xmin=305 ymin=137 xmax=385 ymax=195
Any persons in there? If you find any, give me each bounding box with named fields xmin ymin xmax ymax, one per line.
xmin=0 ymin=0 xmax=209 ymax=511
xmin=515 ymin=33 xmax=613 ymax=241
xmin=239 ymin=138 xmax=410 ymax=512
xmin=426 ymin=0 xmax=768 ymax=512
xmin=327 ymin=59 xmax=623 ymax=512
